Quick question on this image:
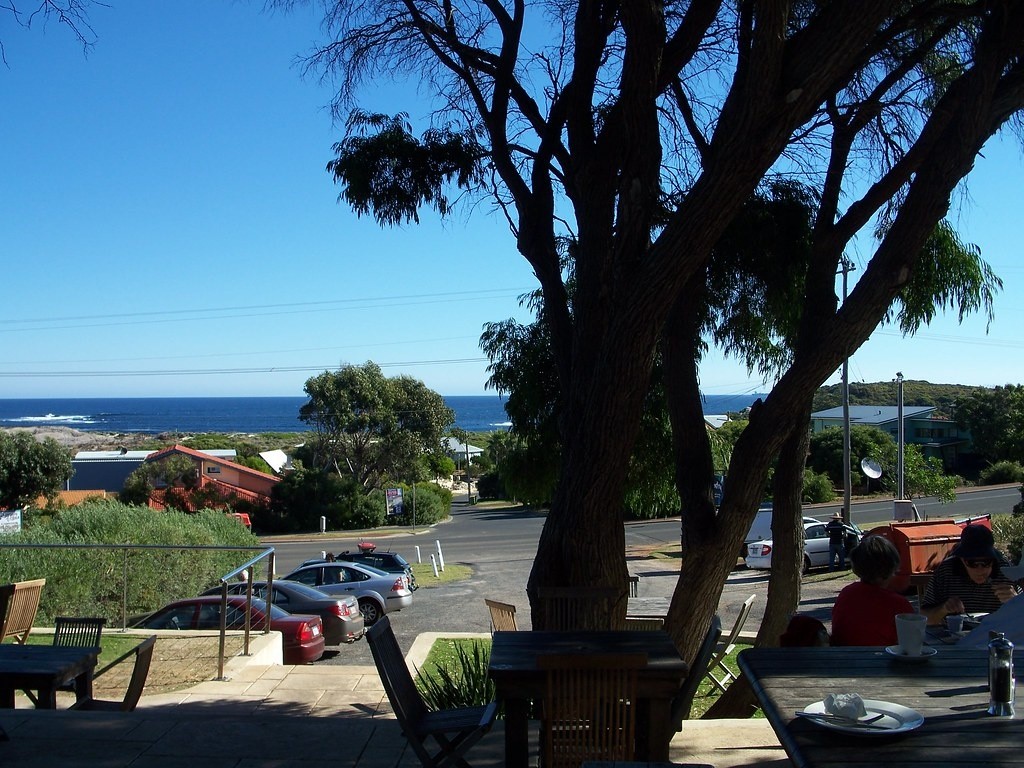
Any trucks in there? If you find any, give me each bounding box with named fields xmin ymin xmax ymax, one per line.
xmin=739 ymin=509 xmax=821 ymax=561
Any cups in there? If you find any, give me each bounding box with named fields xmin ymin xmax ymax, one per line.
xmin=895 ymin=614 xmax=927 ymax=654
xmin=947 ymin=616 xmax=963 ymax=634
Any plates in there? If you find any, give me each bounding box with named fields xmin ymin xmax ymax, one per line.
xmin=804 ymin=699 xmax=924 ymax=733
xmin=886 ymin=646 xmax=937 ymax=660
xmin=958 ymin=613 xmax=990 ymax=626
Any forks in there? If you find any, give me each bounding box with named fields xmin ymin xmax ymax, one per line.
xmin=795 ymin=711 xmax=884 ymax=724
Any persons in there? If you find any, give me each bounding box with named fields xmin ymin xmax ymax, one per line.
xmin=323 ymin=552 xmax=342 ymax=585
xmin=824 ymin=512 xmax=846 ymax=572
xmin=829 ymin=534 xmax=916 ymax=647
xmin=955 ymin=545 xmax=1024 ymax=649
xmin=919 ymin=524 xmax=1023 ymax=626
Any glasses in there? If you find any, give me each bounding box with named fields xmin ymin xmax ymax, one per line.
xmin=963 ymin=558 xmax=992 ymax=569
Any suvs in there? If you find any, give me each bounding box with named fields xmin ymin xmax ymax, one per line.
xmin=290 ymin=550 xmax=419 ymax=593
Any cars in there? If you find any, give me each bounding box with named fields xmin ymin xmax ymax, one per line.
xmin=745 ymin=522 xmax=864 ymax=573
xmin=279 ymin=562 xmax=412 ymax=626
xmin=276 ymin=559 xmax=413 ymax=596
xmin=117 ymin=580 xmax=364 ymax=646
xmin=129 ymin=595 xmax=325 ymax=665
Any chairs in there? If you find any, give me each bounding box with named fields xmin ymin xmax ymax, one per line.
xmin=0 ymin=572 xmax=937 ymax=768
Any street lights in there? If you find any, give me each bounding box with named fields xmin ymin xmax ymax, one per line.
xmin=896 ymin=372 xmax=904 ymax=500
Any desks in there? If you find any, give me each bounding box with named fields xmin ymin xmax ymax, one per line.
xmin=736 ymin=643 xmax=1024 ymax=768
xmin=923 ymin=613 xmax=992 ymax=645
xmin=488 ymin=629 xmax=690 ymax=768
xmin=0 ymin=644 xmax=102 ymax=710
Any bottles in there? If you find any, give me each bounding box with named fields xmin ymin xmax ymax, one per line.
xmin=987 ymin=632 xmax=1016 ymax=717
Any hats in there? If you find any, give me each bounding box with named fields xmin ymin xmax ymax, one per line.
xmin=829 ymin=512 xmax=843 ymax=519
xmin=949 ymin=525 xmax=1004 ymax=560
